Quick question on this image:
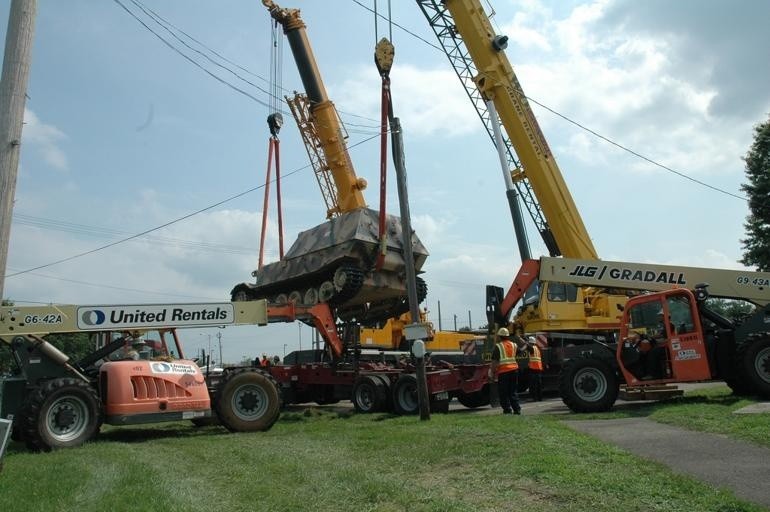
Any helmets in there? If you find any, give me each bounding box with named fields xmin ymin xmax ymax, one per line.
xmin=496 ymin=328 xmax=510 ymax=337
xmin=529 ymin=336 xmax=537 ymax=344
xmin=656 ymin=307 xmax=671 ymax=316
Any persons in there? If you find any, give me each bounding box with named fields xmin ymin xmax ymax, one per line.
xmin=514 ymin=332 xmax=545 ymax=403
xmin=271 ymin=355 xmax=281 ymax=365
xmin=487 ymin=327 xmax=530 ymax=415
xmin=254 ymin=357 xmax=262 ymax=368
xmin=639 ymin=307 xmax=676 ymax=381
xmin=260 ymin=355 xmax=270 ymax=366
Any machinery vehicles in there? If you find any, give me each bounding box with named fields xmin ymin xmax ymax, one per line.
xmin=231 ymin=206 xmax=429 ymax=322
xmin=3 ymin=299 xmax=284 ymax=450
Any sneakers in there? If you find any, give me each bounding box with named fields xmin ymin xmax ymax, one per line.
xmin=503 ymin=410 xmax=521 ymax=416
xmin=526 ymin=397 xmax=542 ymax=402
